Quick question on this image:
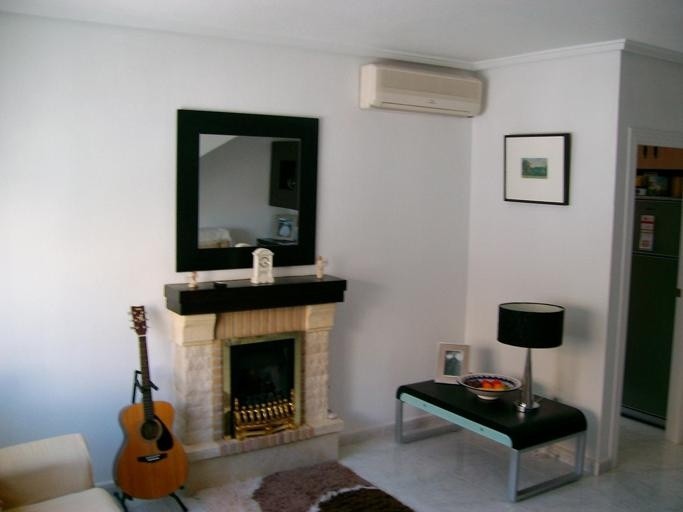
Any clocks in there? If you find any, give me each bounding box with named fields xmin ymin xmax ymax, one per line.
xmin=251 ymin=248 xmax=274 ymax=285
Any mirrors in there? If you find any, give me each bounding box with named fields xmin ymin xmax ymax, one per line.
xmin=176 ymin=108 xmax=318 ymax=272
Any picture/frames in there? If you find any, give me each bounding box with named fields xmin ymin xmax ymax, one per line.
xmin=435 ymin=342 xmax=468 ymax=385
xmin=503 ymin=133 xmax=570 ymax=206
xmin=271 ymin=212 xmax=297 ymax=242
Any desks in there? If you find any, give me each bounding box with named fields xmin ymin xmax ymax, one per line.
xmin=256 ymin=237 xmax=279 ymax=246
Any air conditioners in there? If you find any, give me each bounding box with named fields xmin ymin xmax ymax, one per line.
xmin=359 ymin=61 xmax=483 ymax=118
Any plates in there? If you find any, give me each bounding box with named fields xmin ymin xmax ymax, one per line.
xmin=456 ymin=373 xmax=522 ymax=400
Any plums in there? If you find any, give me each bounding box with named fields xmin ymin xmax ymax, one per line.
xmin=482 ymin=380 xmax=505 ymax=389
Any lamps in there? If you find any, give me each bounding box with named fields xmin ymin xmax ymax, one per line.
xmin=497 ymin=302 xmax=565 ymax=411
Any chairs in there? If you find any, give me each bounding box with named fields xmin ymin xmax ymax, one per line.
xmin=0 ymin=433 xmax=122 ymax=512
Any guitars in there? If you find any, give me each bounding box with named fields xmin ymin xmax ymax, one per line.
xmin=113 ymin=305 xmax=188 ymax=499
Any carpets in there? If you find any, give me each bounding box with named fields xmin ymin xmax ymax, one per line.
xmin=102 ymin=462 xmax=418 ymax=512
